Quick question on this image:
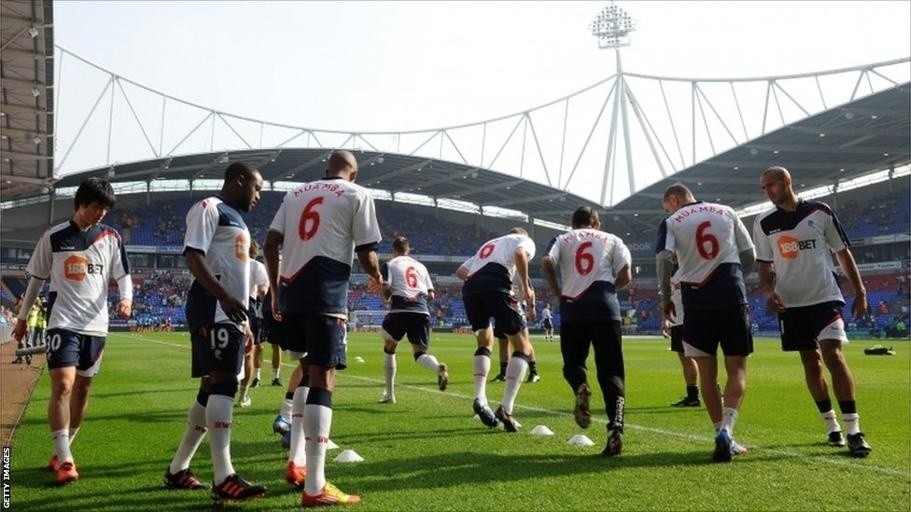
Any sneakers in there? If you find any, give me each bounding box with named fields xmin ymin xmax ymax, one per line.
xmin=490 ymin=375 xmax=507 ymax=384
xmin=473 ymin=397 xmax=500 ymax=427
xmin=713 ymin=428 xmax=747 ymax=461
xmin=573 ymin=383 xmax=592 ymax=429
xmin=437 ymin=362 xmax=448 ymax=392
xmin=828 ymin=431 xmax=845 ymax=447
xmin=238 ymin=377 xmax=281 ymax=409
xmin=272 ymin=415 xmax=361 ymax=506
xmin=600 ymin=428 xmax=622 ymax=458
xmin=379 ymin=394 xmax=396 ymax=405
xmin=494 ymin=405 xmax=519 ymax=433
xmin=526 ymin=373 xmax=540 ymax=383
xmin=847 ymin=432 xmax=872 ymax=458
xmin=12 ymin=358 xmax=23 ymax=364
xmin=50 ymin=452 xmax=78 ymax=484
xmin=671 ymin=396 xmax=700 ymax=407
xmin=163 ymin=466 xmax=211 ymax=490
xmin=210 ymin=473 xmax=266 ymax=502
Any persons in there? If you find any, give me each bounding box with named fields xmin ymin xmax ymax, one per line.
xmin=161 ymin=159 xmax=270 ymax=503
xmin=653 ymin=183 xmax=760 ymax=463
xmin=541 ymin=205 xmax=636 ymax=458
xmin=2 ymin=194 xmax=910 ymax=451
xmin=262 ymin=149 xmax=386 ymax=508
xmin=753 ymin=165 xmax=874 ymax=459
xmin=8 ymin=177 xmax=137 ymax=486
